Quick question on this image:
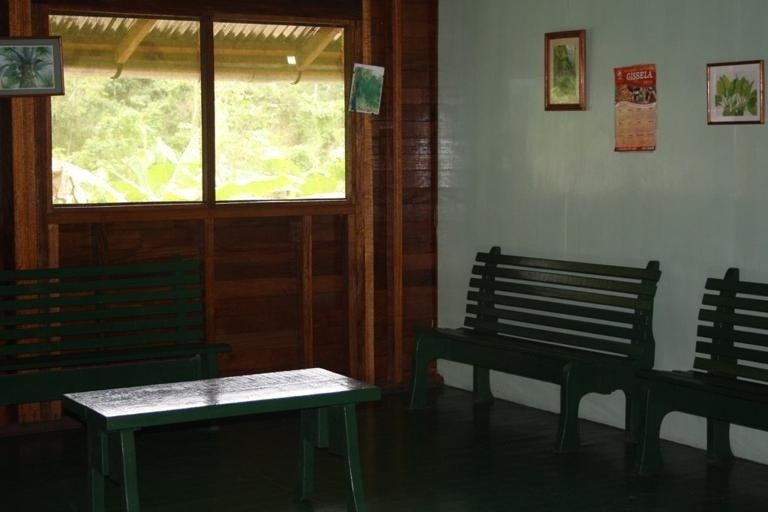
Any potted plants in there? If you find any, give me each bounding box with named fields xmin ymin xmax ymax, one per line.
xmin=0 ymin=35 xmax=65 ymax=98
xmin=706 ymin=60 xmax=765 ymax=126
xmin=543 ymin=29 xmax=588 ymax=112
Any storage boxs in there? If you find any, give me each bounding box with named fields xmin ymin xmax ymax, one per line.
xmin=637 ymin=267 xmax=768 ymax=482
xmin=406 ymin=245 xmax=664 ymax=453
xmin=0 ymin=260 xmax=232 ymax=408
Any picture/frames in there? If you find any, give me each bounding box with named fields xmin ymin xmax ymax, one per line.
xmin=62 ymin=365 xmax=383 ymax=511
